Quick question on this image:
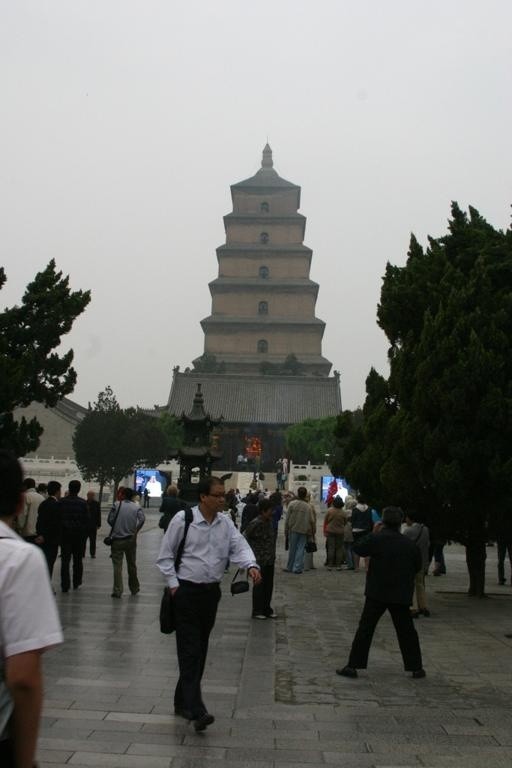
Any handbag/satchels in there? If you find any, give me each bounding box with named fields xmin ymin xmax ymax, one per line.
xmin=104 ymin=537 xmax=112 ymax=545
xmin=159 ymin=587 xmax=178 ymax=634
xmin=159 ymin=514 xmax=172 ymax=528
xmin=285 ymin=535 xmax=289 ymax=551
xmin=306 ymin=542 xmax=317 ymax=553
xmin=231 ymin=582 xmax=249 ymax=594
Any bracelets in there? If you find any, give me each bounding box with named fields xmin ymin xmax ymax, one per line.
xmin=248 ymin=566 xmax=258 ymax=570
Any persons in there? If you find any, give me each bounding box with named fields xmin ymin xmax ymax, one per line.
xmin=155 ymin=477 xmax=262 ymax=731
xmin=403 ymin=513 xmax=433 ymax=617
xmin=336 ymin=507 xmax=425 ymax=678
xmin=225 ymin=487 xmax=371 ymax=574
xmin=240 ymin=501 xmax=278 ymax=619
xmin=1 ymin=478 xmax=150 ymax=598
xmin=0 ymin=453 xmax=64 ymax=767
xmin=497 ymin=539 xmax=511 ymax=584
xmin=159 ymin=486 xmax=176 ymax=534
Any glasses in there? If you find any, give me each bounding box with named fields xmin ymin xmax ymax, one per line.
xmin=207 ymin=492 xmax=225 ymax=498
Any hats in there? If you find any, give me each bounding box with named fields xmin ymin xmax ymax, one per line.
xmin=333 ymin=498 xmax=345 ymax=508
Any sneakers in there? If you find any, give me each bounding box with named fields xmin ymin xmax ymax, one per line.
xmin=324 ymin=561 xmax=353 ymax=571
xmin=252 ymin=613 xmax=278 ymax=619
xmin=412 ymin=608 xmax=431 ymax=618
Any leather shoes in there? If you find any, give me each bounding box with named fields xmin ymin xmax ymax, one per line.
xmin=111 ymin=589 xmax=140 ymax=598
xmin=174 ymin=705 xmax=214 ymax=731
xmin=413 ymin=669 xmax=425 ymax=678
xmin=62 ymin=583 xmax=78 ymax=592
xmin=336 ymin=666 xmax=357 ymax=677
xmin=92 ymin=555 xmax=96 ymax=558
